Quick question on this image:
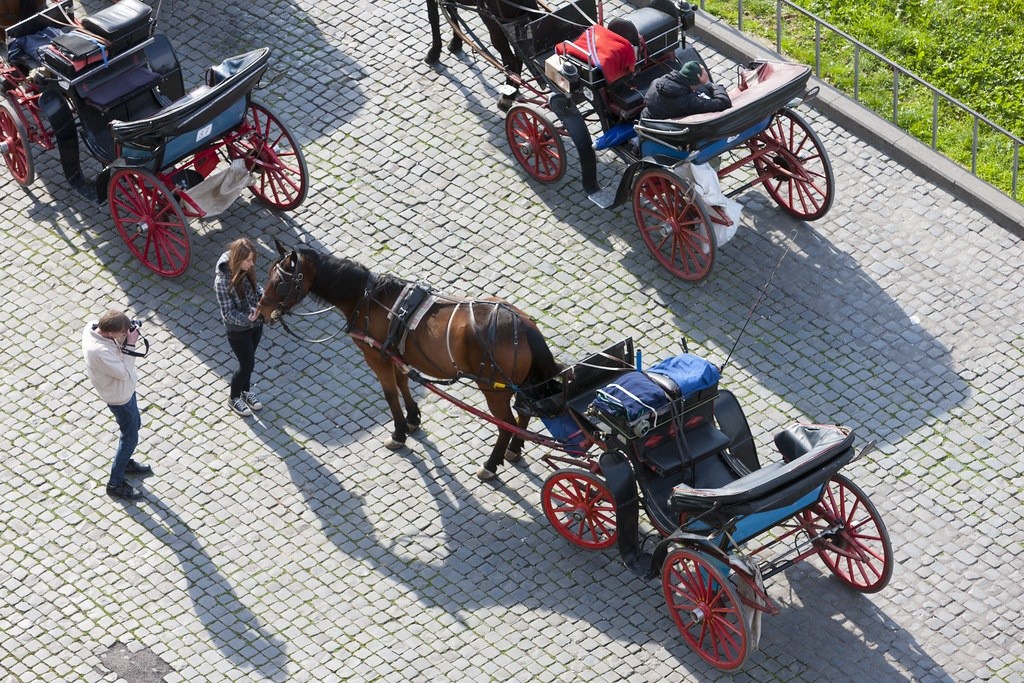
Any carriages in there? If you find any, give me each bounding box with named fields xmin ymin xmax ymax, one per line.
xmin=0 ymin=0 xmax=309 ymax=280
xmin=421 ymin=0 xmax=837 ymax=285
xmin=247 ymin=236 xmax=898 ymax=672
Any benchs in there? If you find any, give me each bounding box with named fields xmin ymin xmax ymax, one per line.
xmin=629 ymin=59 xmax=811 ymax=144
xmin=107 ymin=47 xmax=271 ymax=171
xmin=665 ymin=423 xmax=856 ymax=527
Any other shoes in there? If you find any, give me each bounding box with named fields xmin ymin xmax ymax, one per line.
xmin=124 ymin=459 xmax=150 ymax=473
xmin=106 ymin=481 xmax=142 ymax=500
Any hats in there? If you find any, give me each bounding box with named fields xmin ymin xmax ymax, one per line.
xmin=679 ymin=61 xmax=701 ymax=85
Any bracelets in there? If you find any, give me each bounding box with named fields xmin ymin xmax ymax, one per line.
xmin=126 ymin=343 xmax=135 ymax=347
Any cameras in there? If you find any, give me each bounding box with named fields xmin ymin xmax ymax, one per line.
xmin=130 ymin=320 xmax=142 ymax=332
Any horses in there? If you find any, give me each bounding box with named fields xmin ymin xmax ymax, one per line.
xmin=257 ymin=239 xmax=561 ymax=481
xmin=0 ymin=0 xmax=48 ymax=44
xmin=426 ymin=0 xmax=542 ymax=113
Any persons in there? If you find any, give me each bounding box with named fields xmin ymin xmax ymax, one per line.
xmin=213 ymin=237 xmax=264 ymax=416
xmin=82 ymin=309 xmax=152 ymax=500
xmin=640 ymin=60 xmax=731 ymax=119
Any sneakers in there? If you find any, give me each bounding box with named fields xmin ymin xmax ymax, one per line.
xmin=228 ymin=398 xmax=252 ymax=416
xmin=241 ymin=390 xmax=262 ymax=410
